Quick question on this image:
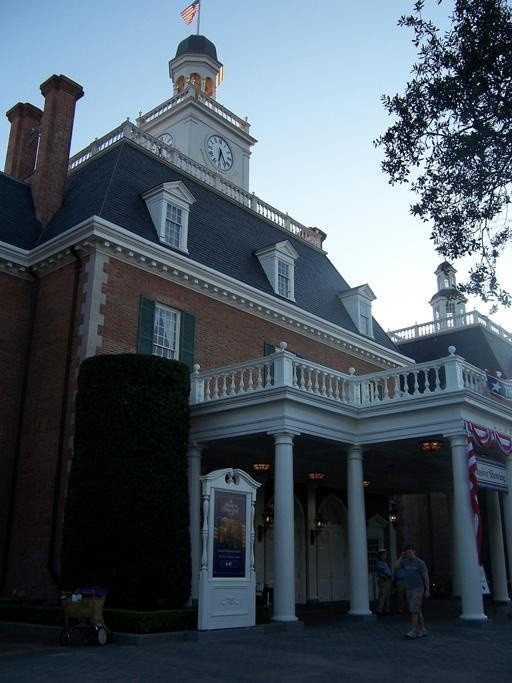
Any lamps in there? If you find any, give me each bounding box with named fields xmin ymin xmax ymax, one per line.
xmin=418 ymin=439 xmax=444 ymax=454
xmin=310 ymin=510 xmax=327 ymax=545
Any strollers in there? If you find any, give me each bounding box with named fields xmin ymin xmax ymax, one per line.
xmin=59 ymin=586 xmax=113 ymax=646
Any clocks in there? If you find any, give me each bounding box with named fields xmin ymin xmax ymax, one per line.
xmin=151 ymin=132 xmax=174 ymax=159
xmin=206 ymin=135 xmax=233 ymax=173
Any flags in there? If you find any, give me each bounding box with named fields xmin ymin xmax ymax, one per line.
xmin=180 ymin=0 xmax=200 ymax=25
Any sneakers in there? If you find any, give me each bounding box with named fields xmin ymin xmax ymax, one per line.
xmin=416 ymin=629 xmax=429 ymax=637
xmin=405 ymin=632 xmax=416 ymax=639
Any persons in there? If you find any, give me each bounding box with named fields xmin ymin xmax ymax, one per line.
xmin=372 ymin=548 xmax=394 ymax=616
xmin=393 ymin=549 xmax=410 ymax=613
xmin=394 ymin=544 xmax=430 ymax=639
